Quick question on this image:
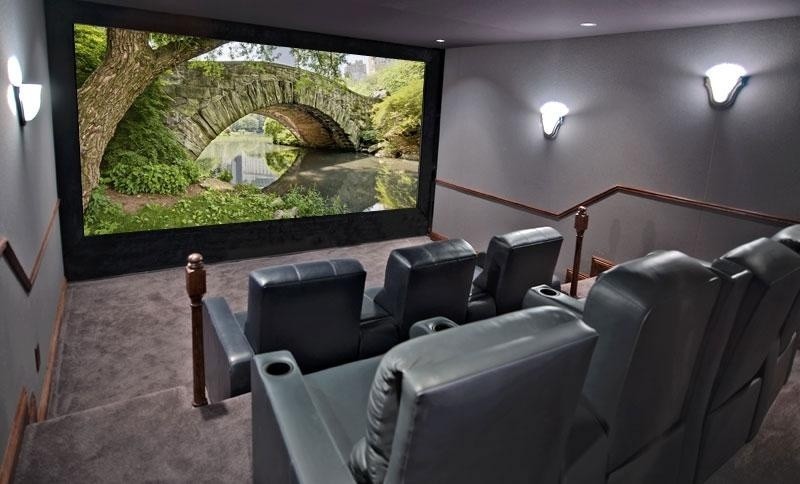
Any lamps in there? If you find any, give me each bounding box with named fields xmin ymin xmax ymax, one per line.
xmin=538 ymin=100 xmax=570 ymax=139
xmin=13 ymin=83 xmax=43 ymax=127
xmin=704 ymin=61 xmax=753 ymax=108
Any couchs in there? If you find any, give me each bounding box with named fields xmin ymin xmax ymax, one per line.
xmin=204 ymin=221 xmax=798 ymax=483
